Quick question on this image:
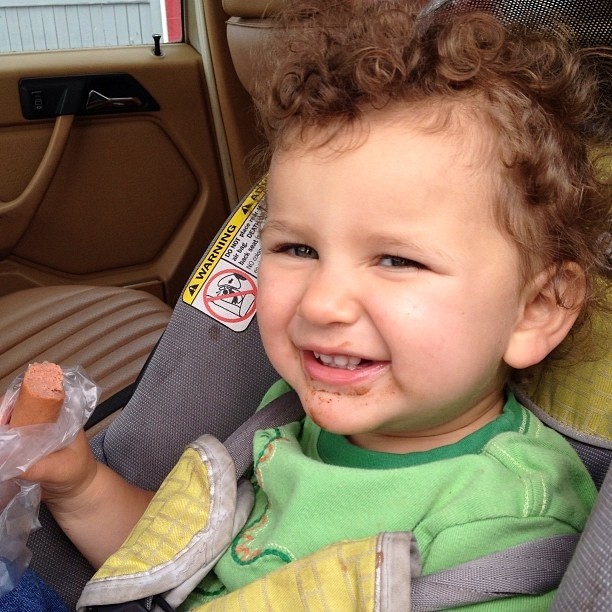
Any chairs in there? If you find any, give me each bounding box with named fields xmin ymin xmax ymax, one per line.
xmin=1 ymin=285 xmax=175 ymax=432
xmin=220 ymin=0 xmax=327 ymax=144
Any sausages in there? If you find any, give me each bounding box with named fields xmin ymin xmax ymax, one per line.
xmin=9 ymin=360 xmax=67 ymax=429
